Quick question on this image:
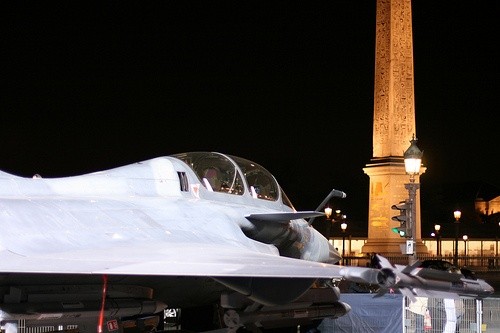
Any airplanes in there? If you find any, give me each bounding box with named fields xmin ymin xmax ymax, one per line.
xmin=0 ymin=150 xmax=494 ymax=333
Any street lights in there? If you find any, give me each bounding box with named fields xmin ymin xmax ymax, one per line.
xmin=435 ymin=223 xmax=441 ymax=261
xmin=453 ymin=209 xmax=461 ymax=266
xmin=462 ymin=233 xmax=468 ymax=266
xmin=324 ymin=204 xmax=348 ymax=266
xmin=403 ymin=133 xmax=423 ymax=266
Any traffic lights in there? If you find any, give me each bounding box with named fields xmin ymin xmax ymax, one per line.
xmin=391 ymin=199 xmax=412 ymax=239
